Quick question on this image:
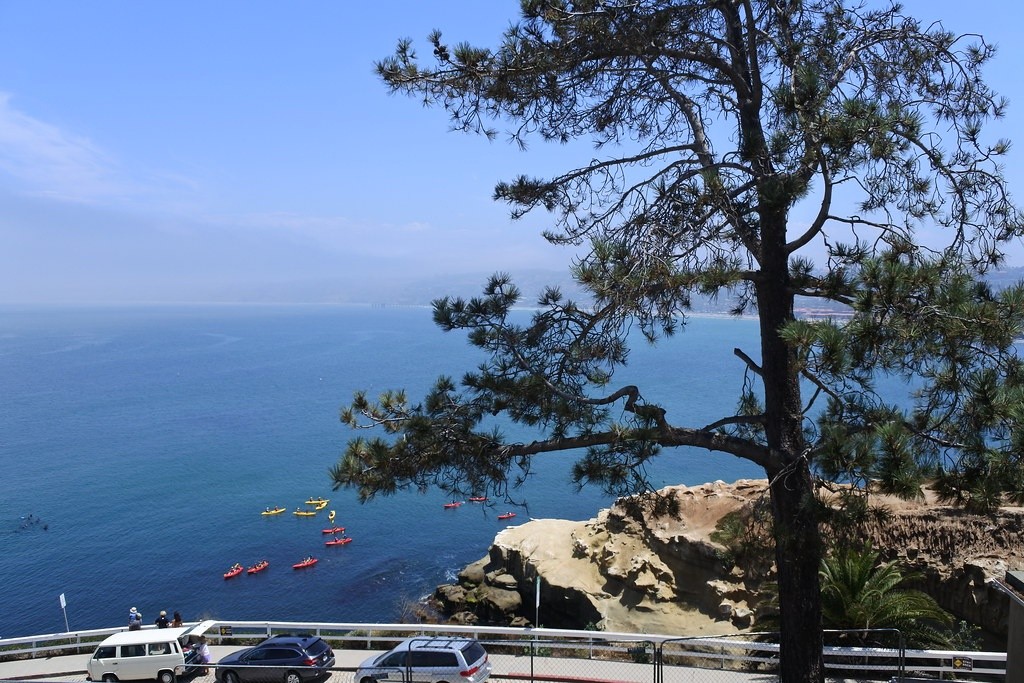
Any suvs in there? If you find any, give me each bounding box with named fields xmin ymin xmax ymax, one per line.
xmin=353 ymin=634 xmax=493 ymax=683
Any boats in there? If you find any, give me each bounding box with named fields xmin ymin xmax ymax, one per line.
xmin=443 ymin=501 xmax=461 ymax=508
xmin=222 ymin=498 xmax=353 ymax=579
xmin=498 ymin=513 xmax=517 ymax=519
xmin=469 ymin=497 xmax=488 ymax=501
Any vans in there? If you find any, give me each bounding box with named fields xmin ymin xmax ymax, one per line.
xmin=85 ymin=620 xmax=217 ymax=683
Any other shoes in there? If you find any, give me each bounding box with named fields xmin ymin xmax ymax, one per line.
xmin=200 ymin=673 xmax=206 ymax=675
xmin=206 ymin=673 xmax=209 ymax=675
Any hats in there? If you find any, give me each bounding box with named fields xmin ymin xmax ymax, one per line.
xmin=130 ymin=607 xmax=137 ymax=613
xmin=160 ymin=611 xmax=166 ymax=616
xmin=136 ymin=613 xmax=141 ymax=617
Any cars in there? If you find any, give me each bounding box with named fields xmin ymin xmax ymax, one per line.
xmin=215 ymin=633 xmax=336 ymax=683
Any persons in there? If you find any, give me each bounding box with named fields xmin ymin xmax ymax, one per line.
xmin=230 ymin=563 xmax=240 ymax=573
xmin=309 ymin=496 xmax=324 ymax=502
xmin=127 ymin=608 xmax=142 ymax=629
xmin=334 ymin=535 xmax=347 ymax=543
xmin=196 ymin=636 xmax=211 ymax=676
xmin=332 ymin=524 xmax=337 ymax=531
xmin=302 ymin=555 xmax=313 ymax=564
xmin=266 ymin=506 xmax=278 ymax=513
xmin=330 ymin=510 xmax=334 ymax=517
xmin=153 ymin=611 xmax=169 ymax=629
xmin=253 ymin=560 xmax=267 ymax=569
xmin=296 ymin=507 xmax=300 ymax=513
xmin=172 ymin=611 xmax=182 ymax=627
